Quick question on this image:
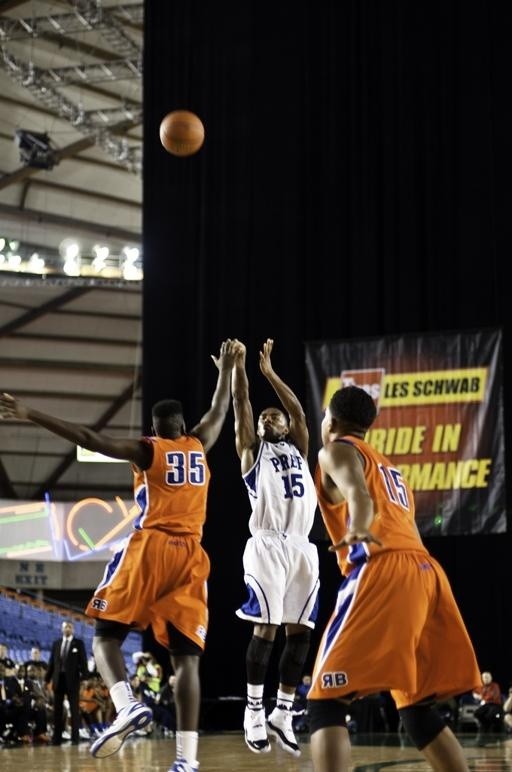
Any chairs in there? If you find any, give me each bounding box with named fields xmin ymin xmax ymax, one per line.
xmin=0 ymin=593 xmax=142 ymax=680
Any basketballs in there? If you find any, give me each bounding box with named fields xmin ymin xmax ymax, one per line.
xmin=160 ymin=110 xmax=205 ymax=156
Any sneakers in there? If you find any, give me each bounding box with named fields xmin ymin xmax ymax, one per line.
xmin=88 ymin=702 xmax=153 ymax=758
xmin=266 ymin=707 xmax=302 ymax=757
xmin=243 ymin=705 xmax=271 ymax=754
xmin=167 ymin=758 xmax=199 ymax=772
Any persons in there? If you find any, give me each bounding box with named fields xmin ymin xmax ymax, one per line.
xmin=0 ymin=335 xmax=244 ymax=772
xmin=501 ymin=687 xmax=512 ymax=729
xmin=301 ymin=379 xmax=485 ymax=772
xmin=471 ymin=670 xmax=503 ymax=748
xmin=296 ymin=674 xmax=312 ymax=714
xmin=0 ymin=615 xmax=174 ymax=750
xmin=226 ymin=332 xmax=326 ymax=762
